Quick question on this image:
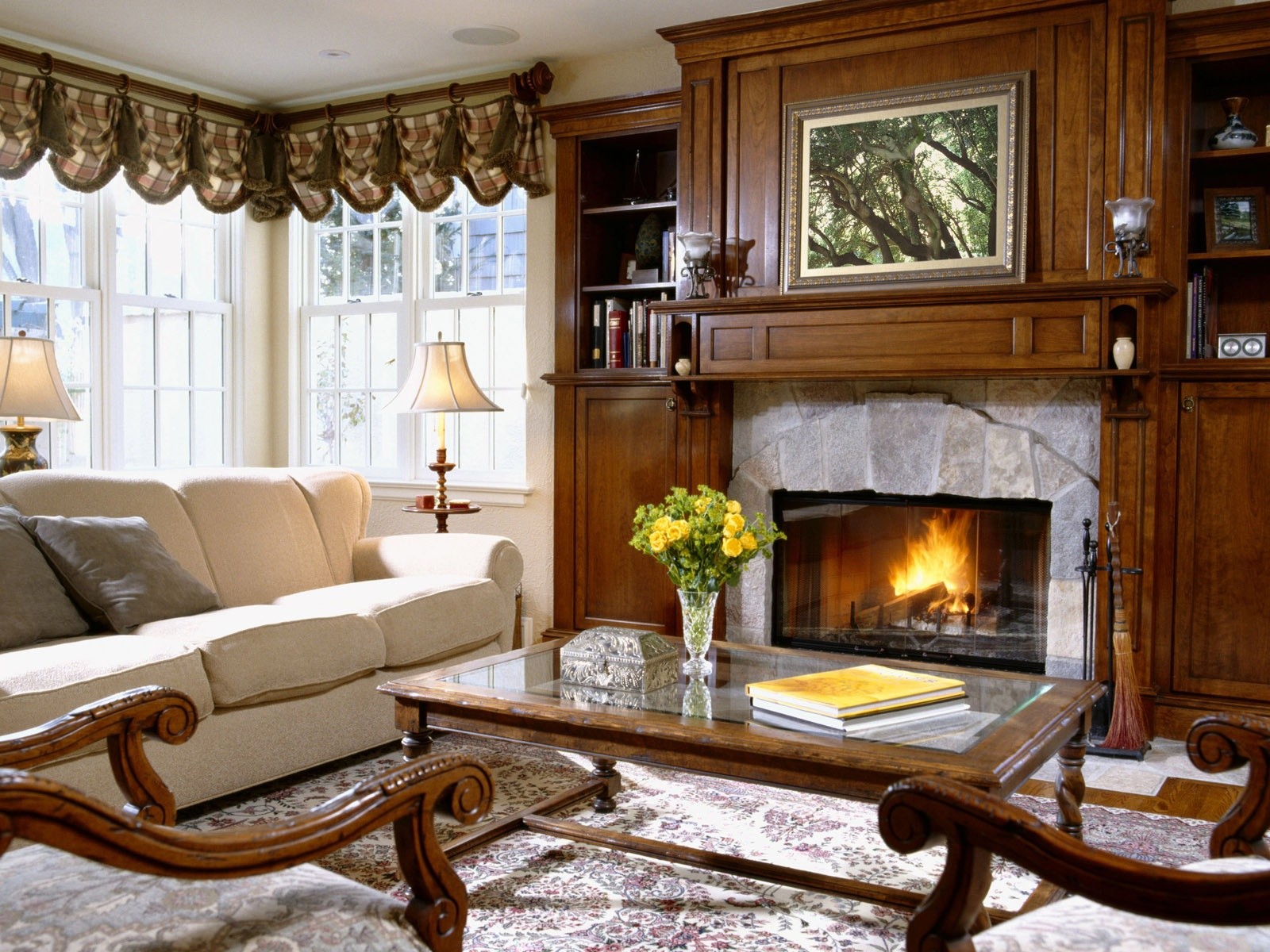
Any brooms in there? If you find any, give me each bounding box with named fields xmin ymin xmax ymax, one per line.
xmin=1094 ymin=533 xmax=1151 ymax=755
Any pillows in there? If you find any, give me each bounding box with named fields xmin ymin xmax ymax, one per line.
xmin=18 ymin=515 xmax=225 ymax=635
xmin=0 ymin=504 xmax=89 ymax=651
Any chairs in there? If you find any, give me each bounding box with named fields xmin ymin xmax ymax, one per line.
xmin=879 ymin=711 xmax=1270 ymax=952
xmin=0 ymin=683 xmax=495 ymax=952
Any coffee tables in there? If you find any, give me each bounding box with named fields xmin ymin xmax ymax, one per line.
xmin=376 ymin=637 xmax=1108 ymax=930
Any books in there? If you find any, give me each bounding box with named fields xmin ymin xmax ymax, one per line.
xmin=662 ymin=231 xmax=676 ymax=282
xmin=1186 ymin=264 xmax=1219 ymax=359
xmin=593 ymin=291 xmax=667 ymax=368
xmin=744 ymin=665 xmax=971 ymax=736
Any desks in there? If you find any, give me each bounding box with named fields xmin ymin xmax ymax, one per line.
xmin=406 ymin=505 xmax=479 ymax=534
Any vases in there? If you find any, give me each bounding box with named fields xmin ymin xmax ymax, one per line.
xmin=1209 ymin=95 xmax=1258 ymax=149
xmin=682 ymin=668 xmax=712 ymax=725
xmin=674 ymin=588 xmax=720 ymax=669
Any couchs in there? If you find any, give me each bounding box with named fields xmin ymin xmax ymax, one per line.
xmin=0 ymin=467 xmax=523 ymax=811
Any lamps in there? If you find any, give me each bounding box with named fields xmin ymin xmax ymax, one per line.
xmin=0 ymin=332 xmax=81 ymax=480
xmin=677 ymin=232 xmax=716 ymax=299
xmin=388 ymin=332 xmax=504 ymax=507
xmin=1104 ymin=197 xmax=1155 ymax=277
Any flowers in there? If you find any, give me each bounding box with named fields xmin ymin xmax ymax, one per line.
xmin=629 ymin=484 xmax=787 ymax=590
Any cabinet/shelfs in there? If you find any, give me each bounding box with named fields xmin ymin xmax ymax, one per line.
xmin=543 ymin=369 xmax=732 ymax=663
xmin=1092 ymin=371 xmax=1270 ymax=745
xmin=530 ymin=89 xmax=682 ymax=379
xmin=1161 ymin=0 xmax=1270 ymax=364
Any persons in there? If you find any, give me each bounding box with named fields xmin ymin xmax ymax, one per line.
xmin=852 ymin=116 xmax=943 ymax=260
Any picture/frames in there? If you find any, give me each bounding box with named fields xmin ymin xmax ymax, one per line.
xmin=778 ymin=71 xmax=1031 ymax=294
xmin=1204 ymin=186 xmax=1270 ymax=252
